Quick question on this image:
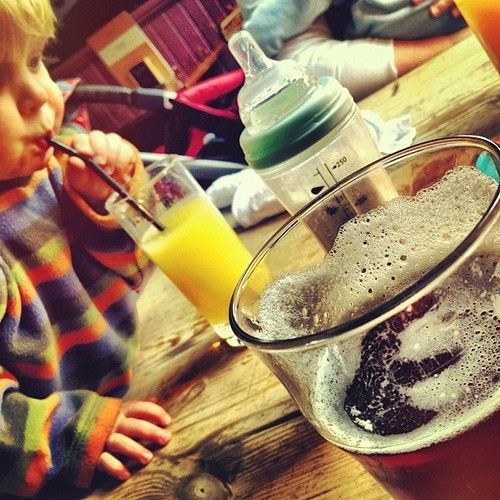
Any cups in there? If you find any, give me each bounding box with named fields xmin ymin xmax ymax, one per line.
xmin=104 ymin=157 xmax=267 ymax=348
xmin=230 ymin=135 xmax=500 ymax=500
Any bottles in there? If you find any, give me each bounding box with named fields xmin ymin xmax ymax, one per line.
xmin=227 ymin=30 xmax=400 ymax=254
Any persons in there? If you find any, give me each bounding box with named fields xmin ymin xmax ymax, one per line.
xmin=0 ymin=0 xmax=171 ymax=500
xmin=236 ymin=0 xmax=473 ymax=103
